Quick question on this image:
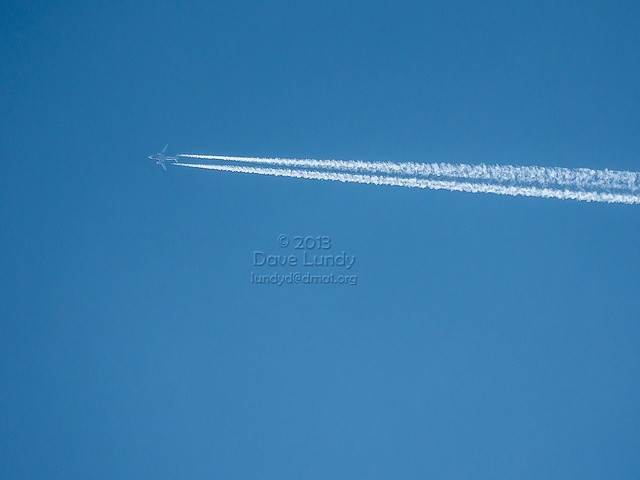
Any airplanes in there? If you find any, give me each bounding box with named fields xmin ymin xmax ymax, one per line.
xmin=148 ymin=145 xmax=180 ymax=171
